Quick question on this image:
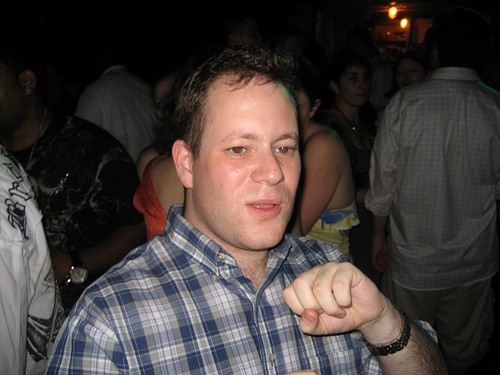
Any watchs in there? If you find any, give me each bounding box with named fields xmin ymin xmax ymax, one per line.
xmin=64 ymin=248 xmax=89 ymax=287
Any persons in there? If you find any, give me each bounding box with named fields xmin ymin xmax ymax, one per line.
xmin=365 ymin=6 xmax=500 ymax=375
xmin=0 ymin=145 xmax=67 ymax=375
xmin=0 ymin=51 xmax=145 ymax=306
xmin=289 ymin=54 xmax=362 ymax=259
xmin=35 ymin=28 xmax=179 ymax=165
xmin=46 ymin=46 xmax=450 ymax=375
xmin=226 ymin=17 xmax=426 ymax=188
xmin=134 ymin=94 xmax=183 ymax=245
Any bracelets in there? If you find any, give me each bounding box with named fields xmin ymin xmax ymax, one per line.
xmin=362 ymin=305 xmax=411 ymax=355
